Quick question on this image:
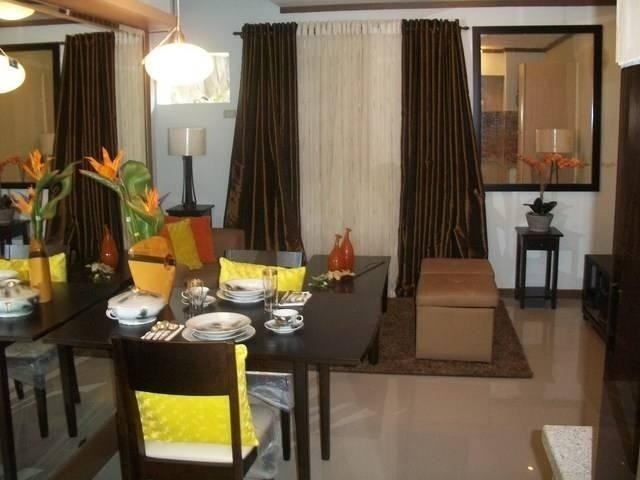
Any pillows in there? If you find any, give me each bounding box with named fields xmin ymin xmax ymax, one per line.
xmin=165 ymin=217 xmax=203 ymax=272
xmin=219 ymin=258 xmax=306 ymax=303
xmin=1 ymin=252 xmax=68 ymax=283
xmin=165 ymin=215 xmax=216 ymax=263
xmin=135 ymin=344 xmax=263 ymax=449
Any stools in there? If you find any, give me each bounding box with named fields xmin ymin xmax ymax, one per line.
xmin=415 ymin=274 xmax=499 ymax=363
xmin=421 ymin=257 xmax=496 ymax=278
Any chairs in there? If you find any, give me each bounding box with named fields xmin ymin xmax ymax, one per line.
xmin=225 ymin=248 xmax=304 ymax=268
xmin=0 ymin=243 xmax=82 ymax=438
xmin=110 ymin=332 xmax=290 ymax=480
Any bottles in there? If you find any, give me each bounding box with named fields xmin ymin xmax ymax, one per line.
xmin=327 ymin=234 xmax=345 ymax=272
xmin=340 ymin=228 xmax=354 ymax=272
xmin=101 ymin=224 xmax=119 ymax=268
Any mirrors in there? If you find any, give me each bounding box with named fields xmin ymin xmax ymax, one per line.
xmin=471 ymin=23 xmax=602 ymax=192
xmin=0 ymin=0 xmax=148 ymax=480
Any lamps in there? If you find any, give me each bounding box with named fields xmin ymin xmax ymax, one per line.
xmin=168 ymin=127 xmax=206 ymax=208
xmin=536 ymin=129 xmax=574 ymax=184
xmin=143 ymin=1 xmax=215 ymax=91
xmin=0 ymin=47 xmax=26 ymax=94
xmin=1 ymin=2 xmax=35 ymax=20
xmin=39 ymin=133 xmax=54 ymax=157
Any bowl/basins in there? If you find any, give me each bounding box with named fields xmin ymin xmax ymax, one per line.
xmin=219 ymin=279 xmax=271 ymax=297
xmin=185 ymin=311 xmax=252 ymax=337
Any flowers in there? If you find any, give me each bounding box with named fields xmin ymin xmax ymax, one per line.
xmin=78 ymin=146 xmax=172 ymax=241
xmin=507 ymin=152 xmax=591 ymax=213
xmin=1 ymin=149 xmax=83 ymax=241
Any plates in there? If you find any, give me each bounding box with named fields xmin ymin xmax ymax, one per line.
xmin=179 ymin=295 xmax=216 ymax=307
xmin=264 ymin=319 xmax=305 ymax=335
xmin=215 ymin=289 xmax=273 ymax=304
xmin=181 ymin=327 xmax=257 ymax=344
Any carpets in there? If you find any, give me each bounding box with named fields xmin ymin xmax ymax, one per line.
xmin=74 ymin=297 xmax=534 ymax=379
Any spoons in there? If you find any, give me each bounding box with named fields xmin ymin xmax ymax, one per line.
xmin=143 ymin=319 xmax=181 ymax=341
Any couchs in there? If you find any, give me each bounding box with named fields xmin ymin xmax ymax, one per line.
xmin=175 ymin=228 xmax=245 ymax=287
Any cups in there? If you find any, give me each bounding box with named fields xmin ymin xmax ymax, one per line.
xmin=187 ymin=278 xmax=204 ymax=311
xmin=272 ymin=309 xmax=304 ymax=328
xmin=181 ymin=287 xmax=210 ymax=301
xmin=262 ymin=268 xmax=279 ymax=313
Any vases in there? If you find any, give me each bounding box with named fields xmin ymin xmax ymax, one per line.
xmin=526 ymin=212 xmax=553 ymax=233
xmin=29 ymin=239 xmax=52 ymax=303
xmin=0 ymin=208 xmax=15 ymax=224
xmin=127 ymin=236 xmax=176 ymax=307
xmin=342 ymin=228 xmax=353 ymax=272
xmin=328 ymin=232 xmax=345 ymax=272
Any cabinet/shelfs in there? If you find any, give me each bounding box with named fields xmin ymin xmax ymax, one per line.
xmin=516 ymin=226 xmax=563 ymax=310
xmin=166 ymin=204 xmax=214 ymax=228
